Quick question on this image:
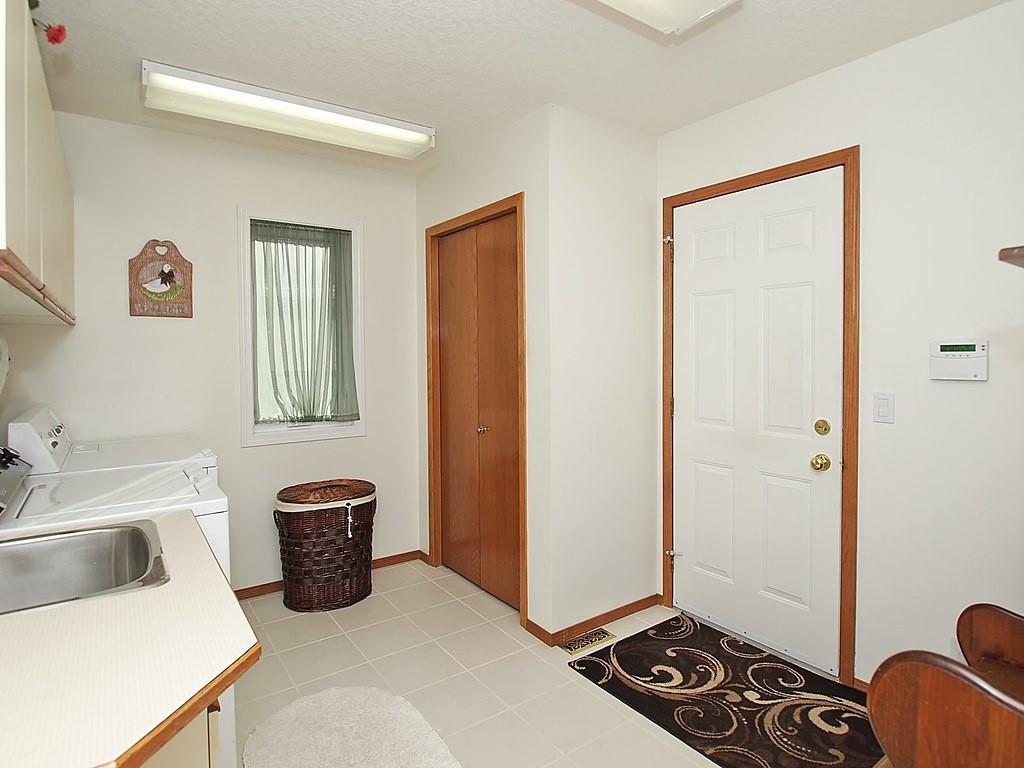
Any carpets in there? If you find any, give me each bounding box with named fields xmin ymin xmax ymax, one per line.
xmin=568 ymin=614 xmax=885 ymax=768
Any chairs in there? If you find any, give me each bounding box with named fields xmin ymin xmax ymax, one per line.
xmin=867 ymin=603 xmax=1024 ymax=768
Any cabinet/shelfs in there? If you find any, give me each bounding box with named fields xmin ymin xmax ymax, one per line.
xmin=0 ymin=0 xmax=77 ymax=327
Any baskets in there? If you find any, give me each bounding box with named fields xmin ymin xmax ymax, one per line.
xmin=273 ymin=478 xmax=377 ymax=613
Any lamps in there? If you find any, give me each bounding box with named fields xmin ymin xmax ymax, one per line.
xmin=140 ymin=57 xmax=436 ymax=160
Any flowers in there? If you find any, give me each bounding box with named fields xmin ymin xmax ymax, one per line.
xmin=28 ymin=0 xmax=68 ymax=45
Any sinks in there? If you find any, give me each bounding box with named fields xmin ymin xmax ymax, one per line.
xmin=0 ymin=519 xmax=171 ymax=615
